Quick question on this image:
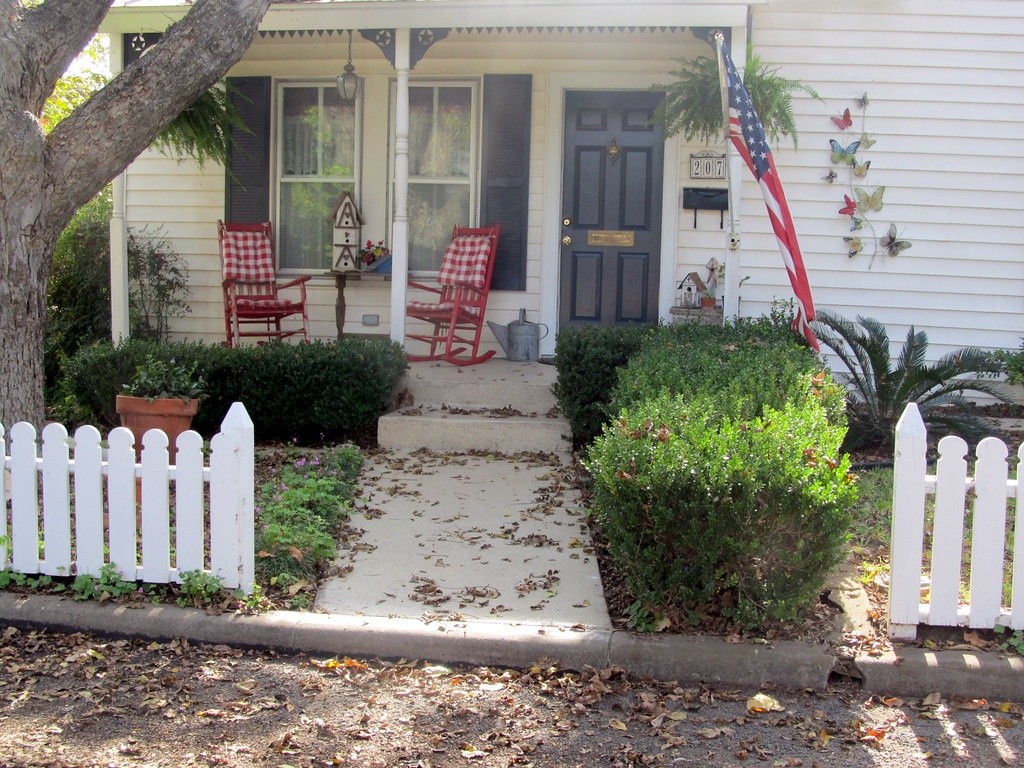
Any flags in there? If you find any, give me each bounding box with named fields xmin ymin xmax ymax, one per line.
xmin=714 ymin=32 xmax=816 ymax=348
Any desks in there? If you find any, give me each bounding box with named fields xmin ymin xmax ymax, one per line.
xmin=324 ymin=272 xmax=413 ymax=342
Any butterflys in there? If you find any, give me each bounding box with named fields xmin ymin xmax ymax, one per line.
xmin=820 ymin=92 xmax=912 ymax=259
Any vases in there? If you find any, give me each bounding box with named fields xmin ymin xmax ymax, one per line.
xmin=367 ymin=254 xmax=392 ymax=272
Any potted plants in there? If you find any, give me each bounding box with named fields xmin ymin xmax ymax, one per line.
xmin=701 ymin=290 xmax=715 ymax=307
xmin=116 ymin=355 xmax=208 ymax=465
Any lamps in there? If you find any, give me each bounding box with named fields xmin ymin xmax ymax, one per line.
xmin=336 ymin=30 xmax=363 ymax=103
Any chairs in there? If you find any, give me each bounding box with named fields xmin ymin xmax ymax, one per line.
xmin=406 ymin=223 xmax=500 ymax=366
xmin=217 ymin=219 xmax=311 ymax=348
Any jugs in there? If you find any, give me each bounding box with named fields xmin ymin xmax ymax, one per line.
xmin=486 ymin=308 xmax=549 ymax=361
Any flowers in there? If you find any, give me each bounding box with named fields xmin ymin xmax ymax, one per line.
xmin=359 ymin=240 xmax=389 ymax=263
